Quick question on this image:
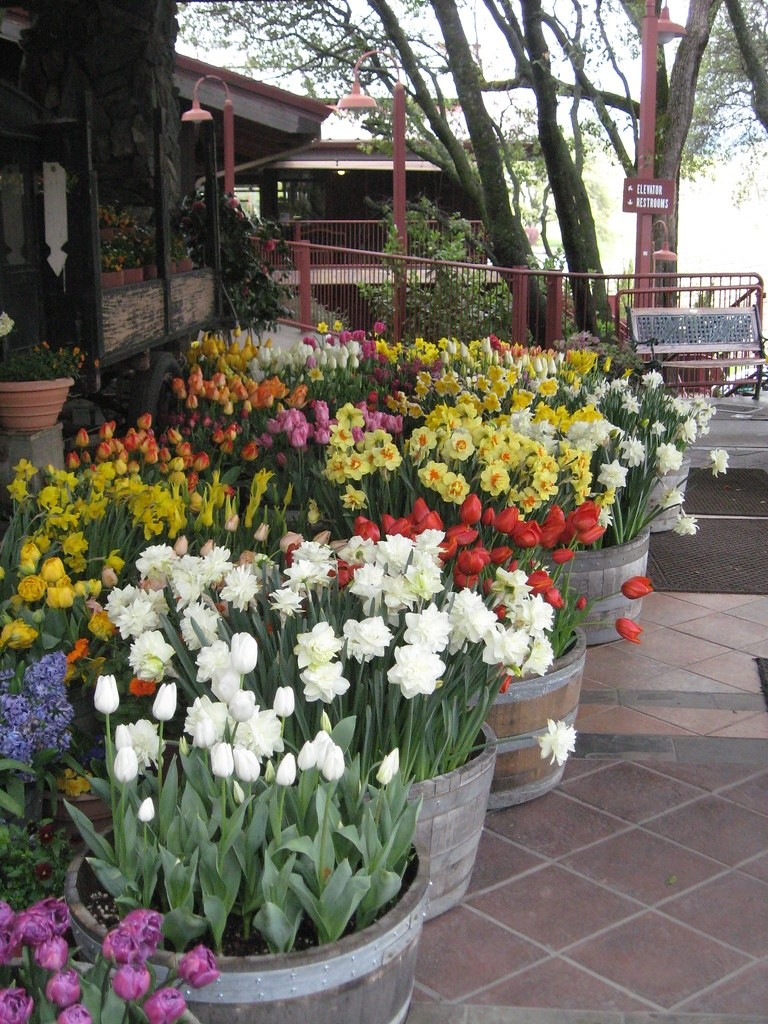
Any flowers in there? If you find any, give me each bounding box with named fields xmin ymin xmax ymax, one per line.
xmin=97 ymin=204 xmax=138 ymax=227
xmin=0 ymin=309 xmax=729 ymax=1024
xmin=99 ymin=227 xmax=188 ymax=272
xmin=176 ymin=191 xmax=288 ymax=341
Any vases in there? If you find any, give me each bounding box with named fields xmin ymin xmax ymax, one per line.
xmin=0 ymin=786 xmax=44 ymax=829
xmin=1 ymin=378 xmax=75 ymax=433
xmin=258 ymin=506 xmax=300 ymax=531
xmin=363 ymin=721 xmax=496 ymax=923
xmin=484 ymin=626 xmax=586 ymax=812
xmin=42 ymin=790 xmax=114 ymax=848
xmin=544 ymin=526 xmax=652 ymax=647
xmin=66 ymin=823 xmax=431 ymax=1024
xmin=648 ymin=456 xmax=692 ymax=533
xmin=101 ymin=256 xmax=193 ymax=290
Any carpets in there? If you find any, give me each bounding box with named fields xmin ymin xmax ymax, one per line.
xmin=684 ymin=464 xmax=768 ymax=516
xmin=645 ymin=518 xmax=768 ymax=595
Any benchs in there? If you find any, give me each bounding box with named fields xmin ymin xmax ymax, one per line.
xmin=616 ymin=285 xmax=768 ymax=401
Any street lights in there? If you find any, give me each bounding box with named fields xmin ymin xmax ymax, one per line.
xmin=650 ymin=218 xmax=677 ymax=307
xmin=181 ymin=75 xmax=236 ymax=207
xmin=334 ymin=50 xmax=410 ymax=344
xmin=632 ymin=0 xmax=689 ymax=384
